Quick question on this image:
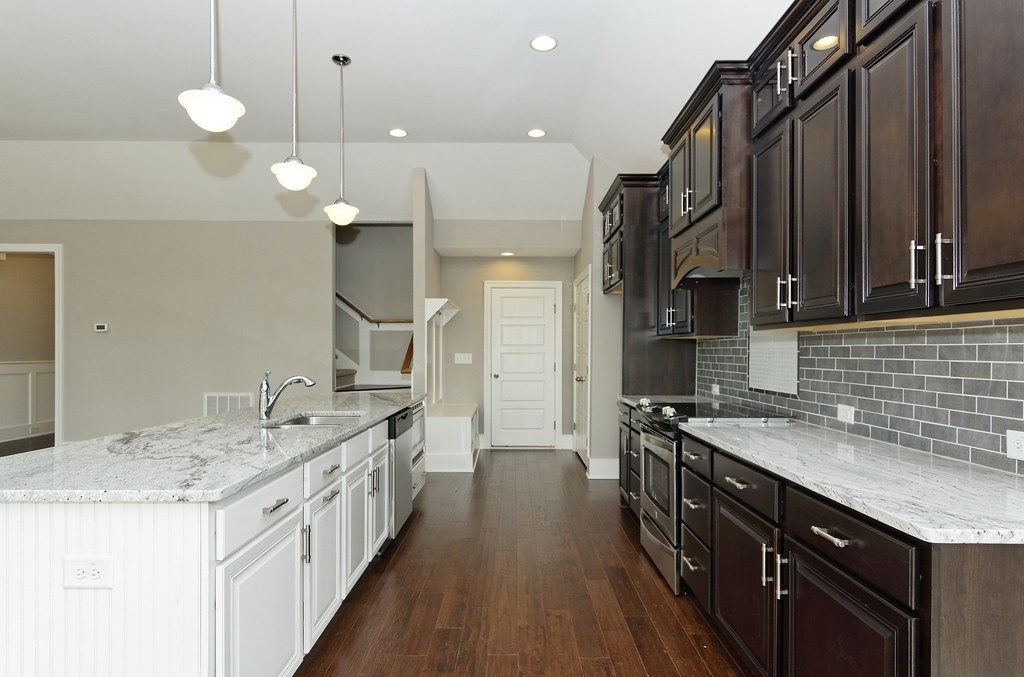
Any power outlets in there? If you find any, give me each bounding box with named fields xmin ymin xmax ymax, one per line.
xmin=1006 ymin=430 xmax=1024 ymax=461
xmin=63 ymin=556 xmax=112 ymax=590
xmin=712 ymin=384 xmax=720 ymax=395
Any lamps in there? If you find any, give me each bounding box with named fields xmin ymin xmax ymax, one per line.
xmin=270 ymin=0 xmax=317 ymax=191
xmin=177 ymin=0 xmax=246 ymax=133
xmin=323 ymin=55 xmax=359 ymax=225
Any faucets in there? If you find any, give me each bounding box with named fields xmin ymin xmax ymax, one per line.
xmin=258 ymin=370 xmax=317 ymax=421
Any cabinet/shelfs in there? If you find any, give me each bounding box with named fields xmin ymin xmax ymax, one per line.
xmin=425 ymin=297 xmax=480 ymax=472
xmin=0 ymin=419 xmax=389 ymax=677
xmin=597 ymin=0 xmax=1023 ymax=676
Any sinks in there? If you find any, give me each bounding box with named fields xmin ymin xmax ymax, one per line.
xmin=252 ymin=411 xmax=366 ymax=430
